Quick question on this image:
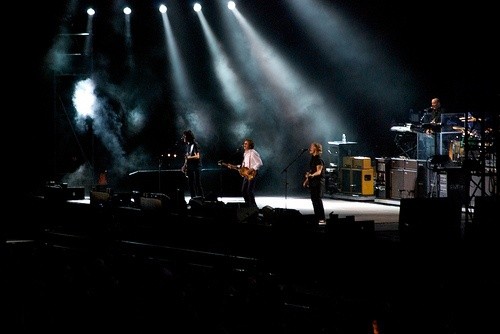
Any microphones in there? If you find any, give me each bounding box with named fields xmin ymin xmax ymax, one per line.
xmin=301 ymin=148 xmax=308 ymax=151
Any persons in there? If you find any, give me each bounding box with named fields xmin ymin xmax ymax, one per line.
xmin=239 ymin=139 xmax=263 ymax=210
xmin=181 ymin=129 xmax=204 ymax=198
xmin=304 ymin=142 xmax=326 ymax=221
xmin=429 ymin=97 xmax=447 ymax=125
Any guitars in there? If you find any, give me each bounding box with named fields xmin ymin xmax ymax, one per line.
xmin=183 ymin=150 xmax=191 ymax=179
xmin=303 ymin=169 xmax=312 ymax=189
xmin=217 ymin=159 xmax=257 ymax=181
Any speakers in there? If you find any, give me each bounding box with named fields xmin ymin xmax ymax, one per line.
xmin=340 ymin=168 xmax=353 ymax=193
xmin=445 ymin=165 xmax=466 ymax=197
xmin=352 ymin=168 xmax=374 ymax=195
xmin=375 ymin=159 xmax=392 ymax=199
xmin=418 ymin=161 xmax=427 ymax=199
xmin=389 ymin=160 xmax=417 ymax=200
